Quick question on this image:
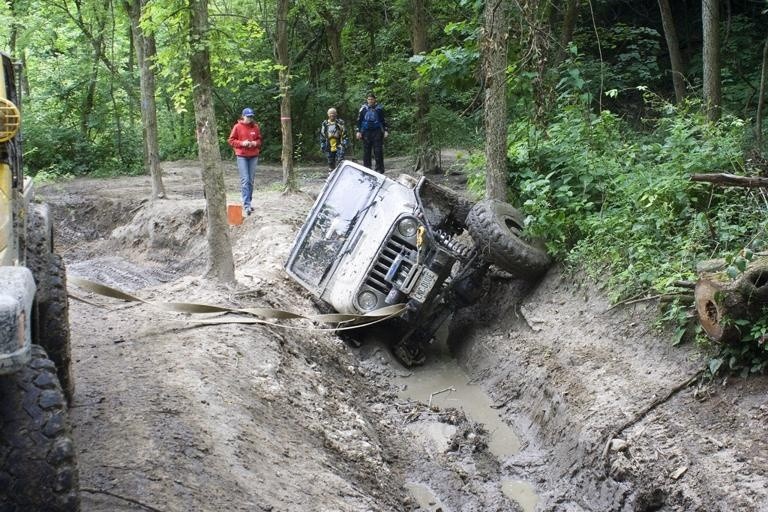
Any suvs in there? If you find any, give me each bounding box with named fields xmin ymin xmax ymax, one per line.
xmin=0 ymin=51 xmax=81 ymax=512
xmin=283 ymin=159 xmax=555 ymax=368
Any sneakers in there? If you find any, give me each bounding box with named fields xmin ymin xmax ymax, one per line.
xmin=246 ymin=206 xmax=254 ymax=215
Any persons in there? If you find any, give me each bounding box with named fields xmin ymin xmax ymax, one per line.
xmin=319 ymin=107 xmax=349 ymax=174
xmin=227 ymin=108 xmax=262 ymax=218
xmin=354 ymin=94 xmax=389 ymax=176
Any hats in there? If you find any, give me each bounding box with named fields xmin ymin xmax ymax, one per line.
xmin=243 ymin=107 xmax=255 ymax=117
